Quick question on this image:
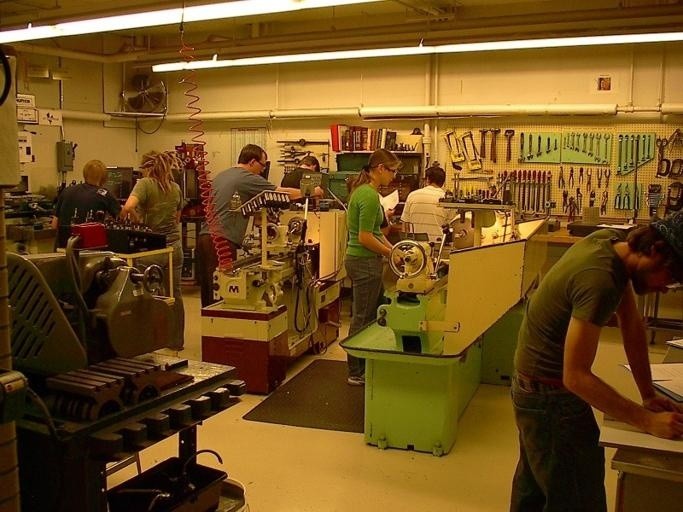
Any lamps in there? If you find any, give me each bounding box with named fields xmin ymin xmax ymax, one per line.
xmin=153 ymin=27 xmax=683 ymax=72
xmin=0 ymin=0 xmax=385 ymax=47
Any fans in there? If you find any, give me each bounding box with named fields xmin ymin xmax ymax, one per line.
xmin=122 ymin=68 xmax=166 ymax=112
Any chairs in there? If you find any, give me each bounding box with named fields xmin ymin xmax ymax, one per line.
xmin=611 ymin=338 xmax=683 ymax=512
xmin=68 ymin=222 xmax=109 ymax=250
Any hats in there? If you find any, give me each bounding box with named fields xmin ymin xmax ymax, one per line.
xmin=650 ymin=210 xmax=683 ymax=258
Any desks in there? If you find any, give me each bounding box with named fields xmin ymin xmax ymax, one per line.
xmin=6 ymin=223 xmax=57 ymax=253
xmin=537 ymin=229 xmax=586 ymax=265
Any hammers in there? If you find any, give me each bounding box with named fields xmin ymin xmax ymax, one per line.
xmin=479 ymin=127 xmax=500 ymax=163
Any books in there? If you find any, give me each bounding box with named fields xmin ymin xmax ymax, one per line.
xmin=376 ymin=189 xmax=399 ymax=213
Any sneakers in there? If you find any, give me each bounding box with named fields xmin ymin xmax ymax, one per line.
xmin=346 ymin=376 xmax=365 ymax=385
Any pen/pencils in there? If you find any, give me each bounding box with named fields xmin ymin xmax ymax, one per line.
xmin=667 ymin=399 xmax=681 ymax=414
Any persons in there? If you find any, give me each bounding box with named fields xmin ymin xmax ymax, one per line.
xmin=281 ymin=155 xmax=320 ymax=211
xmin=49 ymin=158 xmax=120 ymax=251
xmin=197 ymin=143 xmax=324 ymax=308
xmin=502 ymin=208 xmax=683 ymax=512
xmin=361 ymin=171 xmax=395 ymax=239
xmin=342 ymin=146 xmax=406 ymax=388
xmin=117 ymin=149 xmax=185 ymax=351
xmin=398 ymin=165 xmax=457 ymax=244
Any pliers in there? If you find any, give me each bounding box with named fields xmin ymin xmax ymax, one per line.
xmin=557 ymin=167 xmax=609 ymax=214
xmin=280 ymin=150 xmax=306 ymax=158
xmin=614 ymin=185 xmax=639 ymax=210
xmin=656 ymin=139 xmax=668 ymax=158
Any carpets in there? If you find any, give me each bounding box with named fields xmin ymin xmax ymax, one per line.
xmin=241 ymin=359 xmax=364 ymax=435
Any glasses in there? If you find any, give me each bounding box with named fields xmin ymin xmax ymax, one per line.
xmin=667 ymin=264 xmax=682 ymax=293
xmin=256 ymin=159 xmax=266 ymax=170
xmin=386 ymin=167 xmax=398 ymax=177
